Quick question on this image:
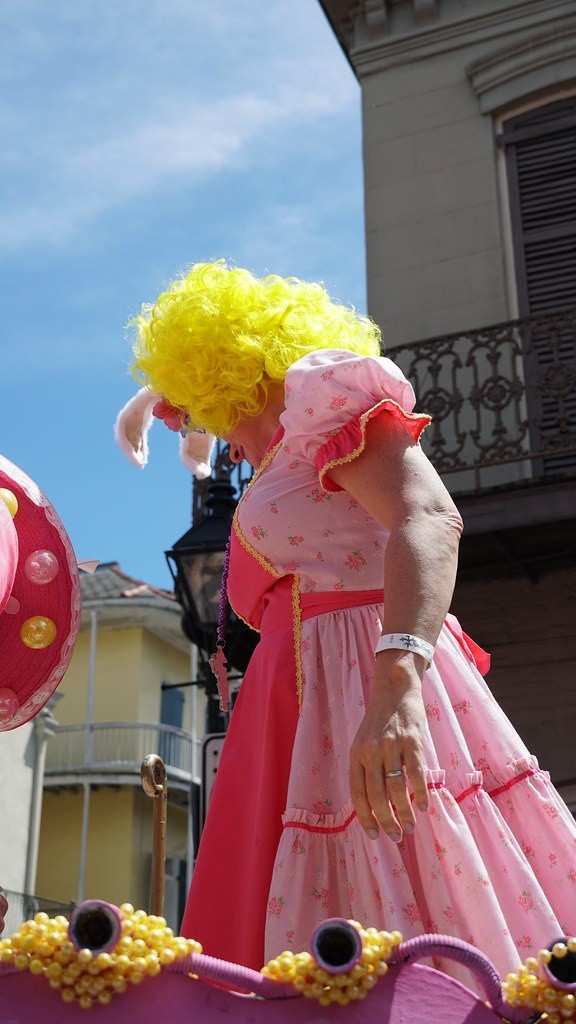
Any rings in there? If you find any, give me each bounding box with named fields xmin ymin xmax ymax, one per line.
xmin=386 ymin=770 xmax=404 ymax=778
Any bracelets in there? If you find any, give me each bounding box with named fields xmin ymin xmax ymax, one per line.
xmin=375 ymin=633 xmax=435 ymax=669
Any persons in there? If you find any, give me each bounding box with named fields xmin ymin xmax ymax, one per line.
xmin=116 ymin=264 xmax=575 ymax=983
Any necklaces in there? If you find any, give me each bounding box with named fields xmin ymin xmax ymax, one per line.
xmin=211 ymin=536 xmax=231 ymax=710
